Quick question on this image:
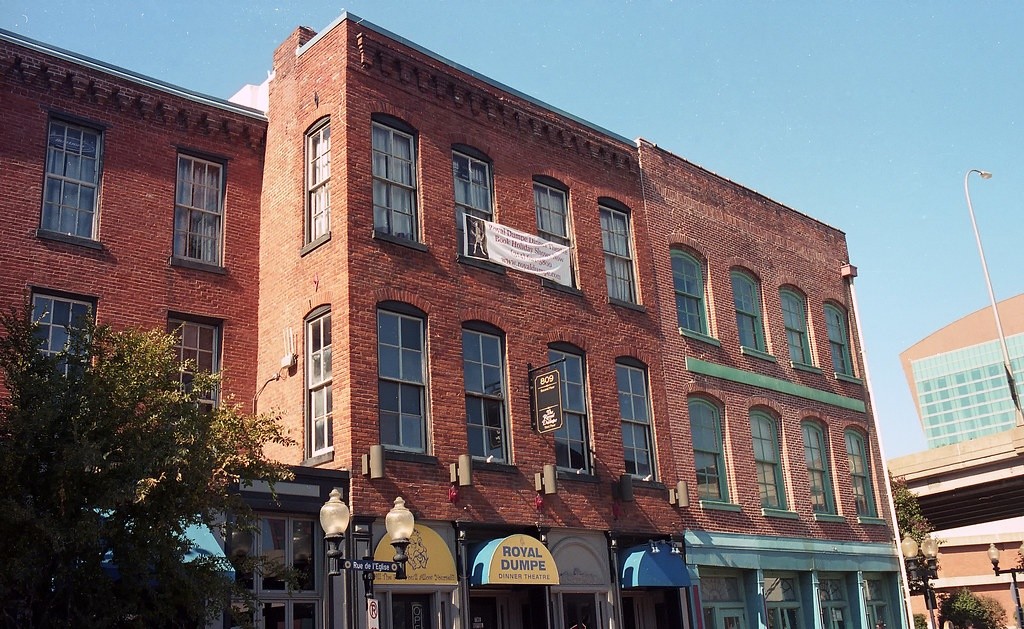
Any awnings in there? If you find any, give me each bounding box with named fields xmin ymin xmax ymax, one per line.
xmin=621 ymin=544 xmax=701 ymax=589
xmin=93 ymin=508 xmax=235 ymax=581
xmin=471 ymin=533 xmax=560 ymax=588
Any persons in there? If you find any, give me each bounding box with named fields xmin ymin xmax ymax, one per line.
xmin=470 ymin=221 xmax=487 ymax=255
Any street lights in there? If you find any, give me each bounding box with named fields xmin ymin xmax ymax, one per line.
xmin=319 ymin=488 xmax=414 ymax=629
xmin=964 ymin=169 xmax=1024 ymax=427
xmin=901 ymin=532 xmax=939 ymax=629
xmin=987 ymin=539 xmax=1024 ymax=629
